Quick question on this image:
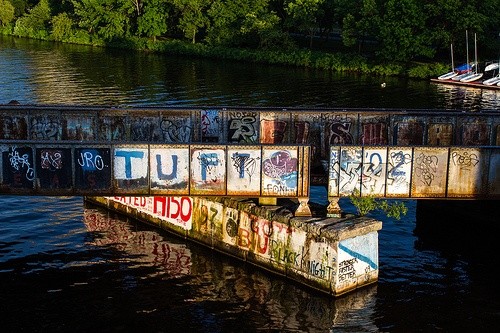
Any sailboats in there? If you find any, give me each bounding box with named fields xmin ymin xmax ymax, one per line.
xmin=459 ymin=33 xmax=483 ymax=82
xmin=438 ymin=42 xmax=458 ymax=80
xmin=483 ymin=73 xmax=500 ymax=85
xmin=451 ymin=30 xmax=474 ymax=81
